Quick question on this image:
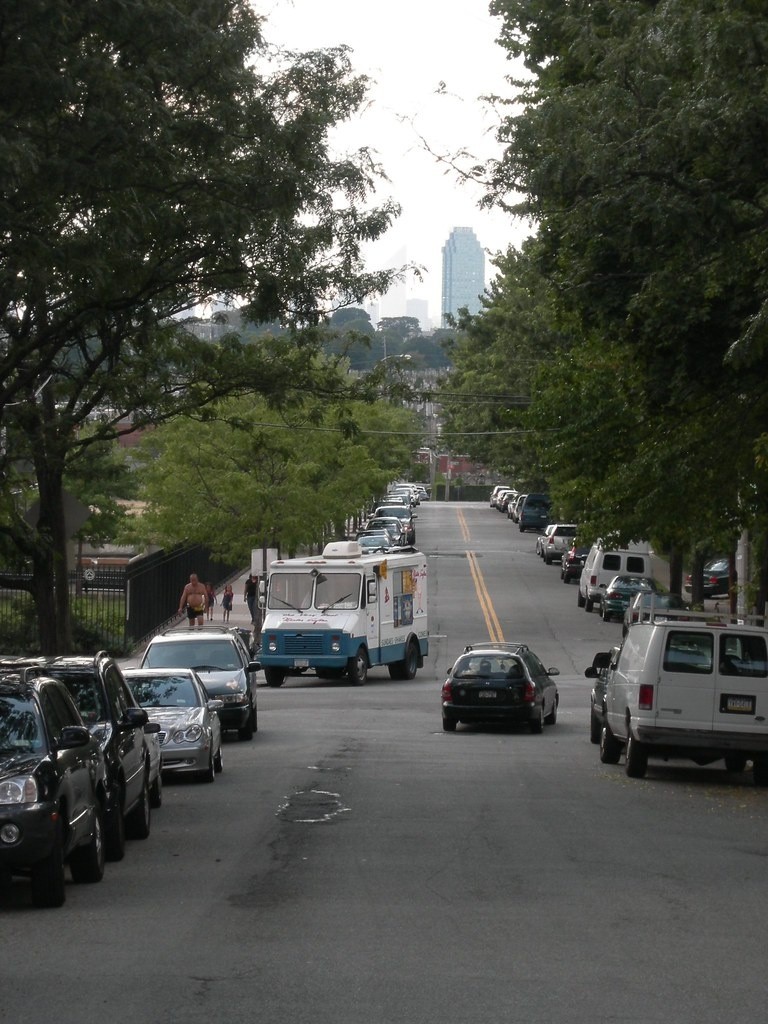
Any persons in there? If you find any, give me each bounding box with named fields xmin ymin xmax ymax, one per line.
xmin=244 ymin=573 xmax=258 ymax=623
xmin=220 ymin=584 xmax=234 ymax=622
xmin=178 ymin=574 xmax=208 ymax=630
xmin=204 ymin=584 xmax=217 ymax=620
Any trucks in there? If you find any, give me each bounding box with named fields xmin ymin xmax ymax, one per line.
xmin=260 ymin=540 xmax=430 ymax=689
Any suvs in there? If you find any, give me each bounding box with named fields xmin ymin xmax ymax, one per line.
xmin=0 ymin=662 xmax=125 ymax=909
xmin=541 ymin=522 xmax=584 ymax=565
xmin=366 ymin=505 xmax=418 ymax=544
xmin=560 ymin=535 xmax=591 ymax=584
xmin=0 ymin=651 xmax=164 ymax=841
xmin=488 ymin=484 xmax=557 ymax=533
xmin=127 ymin=625 xmax=261 ymax=742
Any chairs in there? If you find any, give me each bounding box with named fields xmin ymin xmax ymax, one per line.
xmin=479 ymin=660 xmax=491 ymax=674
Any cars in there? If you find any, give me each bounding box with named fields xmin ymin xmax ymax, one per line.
xmin=441 ymin=641 xmax=560 ymax=735
xmin=355 ymin=528 xmax=396 ymax=548
xmin=364 ymin=516 xmax=408 ymax=546
xmin=585 ymin=665 xmax=608 ymax=745
xmin=536 ymin=524 xmax=554 ymax=556
xmin=686 ymin=555 xmax=736 ymax=599
xmin=599 ymin=574 xmax=663 ymax=620
xmin=375 ymin=480 xmax=430 ymax=511
xmin=120 ymin=666 xmax=224 ymax=782
xmin=621 ymin=588 xmax=689 ymax=639
xmin=356 ymin=534 xmax=394 ymax=556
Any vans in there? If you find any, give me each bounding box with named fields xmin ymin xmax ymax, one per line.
xmin=578 ymin=543 xmax=657 ymax=612
xmin=591 ymin=587 xmax=768 ymax=789
